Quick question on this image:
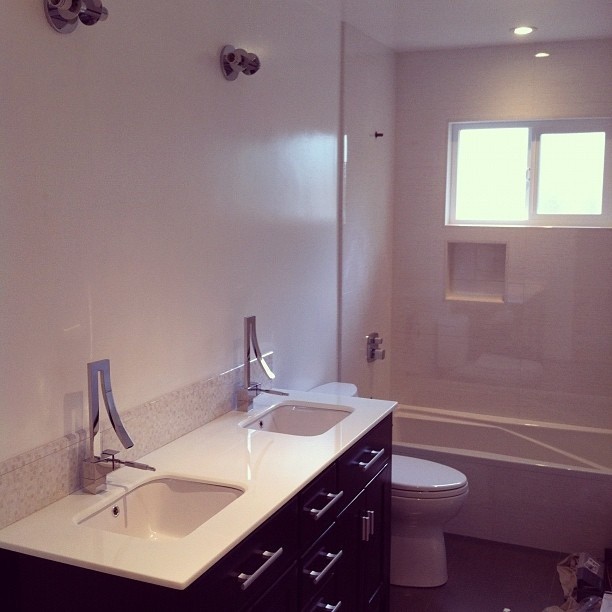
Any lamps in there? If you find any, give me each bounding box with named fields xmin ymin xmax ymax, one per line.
xmin=42 ymin=0 xmax=110 ymax=34
xmin=219 ymin=44 xmax=260 ymax=81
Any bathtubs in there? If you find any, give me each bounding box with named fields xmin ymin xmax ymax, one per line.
xmin=391 ymin=401 xmax=611 ymax=561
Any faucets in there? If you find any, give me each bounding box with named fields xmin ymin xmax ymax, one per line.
xmin=242 ymin=314 xmax=277 ymax=389
xmin=86 ymin=358 xmax=134 ymax=459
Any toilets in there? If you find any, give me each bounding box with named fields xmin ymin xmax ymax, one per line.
xmin=306 ymin=382 xmax=470 ymax=588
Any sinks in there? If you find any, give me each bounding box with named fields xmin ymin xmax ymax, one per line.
xmin=75 ymin=472 xmax=244 ymax=540
xmin=238 ymin=398 xmax=355 ymax=437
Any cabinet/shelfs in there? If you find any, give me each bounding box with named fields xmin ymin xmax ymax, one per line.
xmin=301 ymin=446 xmax=352 ymax=612
xmin=1 ymin=491 xmax=300 ymax=612
xmin=337 ymin=411 xmax=394 ymax=612
